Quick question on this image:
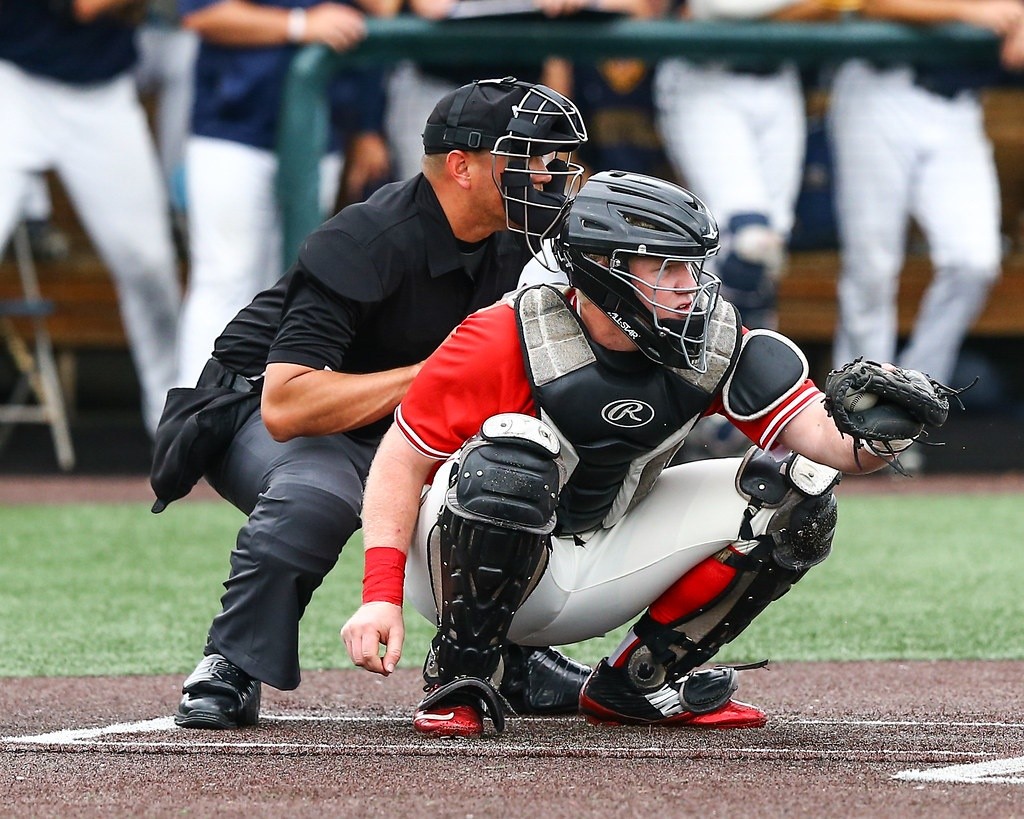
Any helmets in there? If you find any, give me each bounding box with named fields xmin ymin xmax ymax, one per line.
xmin=551 ymin=169 xmax=721 ymax=373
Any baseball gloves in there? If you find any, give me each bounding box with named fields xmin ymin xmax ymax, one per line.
xmin=819 ymin=354 xmax=981 ymax=479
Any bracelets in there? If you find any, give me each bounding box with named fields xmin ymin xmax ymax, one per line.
xmin=286 ymin=5 xmax=307 ymax=44
xmin=362 ymin=548 xmax=406 ymax=607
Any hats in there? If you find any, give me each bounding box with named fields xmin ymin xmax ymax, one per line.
xmin=422 ymin=77 xmax=534 ymax=153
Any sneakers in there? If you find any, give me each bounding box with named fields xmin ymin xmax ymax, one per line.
xmin=499 ymin=645 xmax=595 ymax=717
xmin=413 ymin=677 xmax=485 ymax=738
xmin=174 ymin=653 xmax=261 ymax=730
xmin=578 ymin=654 xmax=767 ymax=729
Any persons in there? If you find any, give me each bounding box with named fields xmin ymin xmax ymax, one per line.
xmin=824 ymin=0 xmax=1024 ymax=471
xmin=174 ymin=77 xmax=588 ymax=729
xmin=654 ymin=0 xmax=839 ymax=336
xmin=0 ymin=0 xmax=594 ymax=442
xmin=339 ymin=170 xmax=950 ymax=740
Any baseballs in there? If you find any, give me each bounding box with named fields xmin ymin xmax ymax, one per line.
xmin=843 ymin=387 xmax=878 ymax=412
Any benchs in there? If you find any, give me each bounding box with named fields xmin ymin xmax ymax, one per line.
xmin=0 ymin=79 xmax=1024 ymax=468
